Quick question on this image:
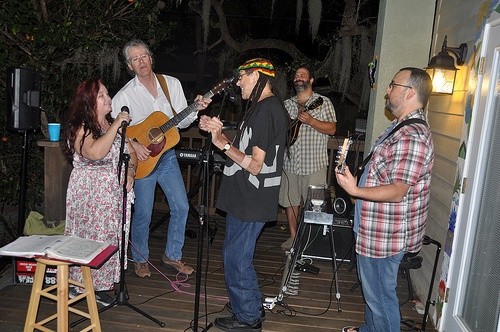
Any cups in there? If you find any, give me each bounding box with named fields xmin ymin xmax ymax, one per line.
xmin=48 ymin=124 xmax=61 ymax=142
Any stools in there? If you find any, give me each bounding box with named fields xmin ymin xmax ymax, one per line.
xmin=24 ymin=259 xmax=102 ymax=332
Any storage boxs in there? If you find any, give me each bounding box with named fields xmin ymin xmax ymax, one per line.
xmin=13 ymin=257 xmax=58 ymax=285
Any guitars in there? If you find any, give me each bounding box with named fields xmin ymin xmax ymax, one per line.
xmin=121 ymin=75 xmax=236 ymax=180
xmin=334 ymin=138 xmax=356 ymax=204
xmin=288 ymin=96 xmax=324 ymax=148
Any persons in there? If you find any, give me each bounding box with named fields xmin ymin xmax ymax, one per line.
xmin=63 ymin=79 xmax=137 ymax=309
xmin=199 ymin=57 xmax=288 ymax=332
xmin=280 ymin=66 xmax=337 ymax=251
xmin=110 ymin=39 xmax=212 ymax=279
xmin=336 ymin=67 xmax=433 ymax=332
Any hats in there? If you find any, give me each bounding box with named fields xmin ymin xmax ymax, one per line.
xmin=237 ymin=58 xmax=275 ymax=77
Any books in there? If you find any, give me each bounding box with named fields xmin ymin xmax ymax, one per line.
xmin=0 ymin=235 xmax=119 ymax=269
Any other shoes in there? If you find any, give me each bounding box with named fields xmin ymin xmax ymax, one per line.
xmin=95 ymin=292 xmax=117 ymax=307
xmin=342 ymin=326 xmax=359 ymax=332
xmin=281 ymin=238 xmax=294 ymax=249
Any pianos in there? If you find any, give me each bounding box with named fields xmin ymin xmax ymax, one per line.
xmin=147 ymin=146 xmax=227 ymax=248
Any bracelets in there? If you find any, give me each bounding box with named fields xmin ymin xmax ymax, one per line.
xmin=129 ymin=167 xmax=136 ymax=177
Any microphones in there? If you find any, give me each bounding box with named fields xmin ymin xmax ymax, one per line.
xmin=121 ymin=106 xmax=129 ymax=125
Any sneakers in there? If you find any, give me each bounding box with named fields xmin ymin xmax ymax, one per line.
xmin=134 ymin=263 xmax=151 ymax=278
xmin=161 ymin=254 xmax=194 ymax=275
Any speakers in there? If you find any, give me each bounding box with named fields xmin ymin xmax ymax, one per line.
xmin=7 ymin=66 xmax=43 ymax=133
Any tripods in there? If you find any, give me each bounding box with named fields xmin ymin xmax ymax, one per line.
xmin=70 ymin=125 xmax=165 ymax=328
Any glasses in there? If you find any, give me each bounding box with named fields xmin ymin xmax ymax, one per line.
xmin=128 ymin=54 xmax=149 ymax=63
xmin=389 ymin=83 xmax=413 ymax=89
xmin=237 ymin=72 xmax=253 ymax=80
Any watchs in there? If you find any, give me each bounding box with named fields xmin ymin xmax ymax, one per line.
xmin=220 ymin=142 xmax=231 ymax=153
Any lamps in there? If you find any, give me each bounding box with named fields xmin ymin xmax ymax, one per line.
xmin=425 ymin=35 xmax=468 ymax=95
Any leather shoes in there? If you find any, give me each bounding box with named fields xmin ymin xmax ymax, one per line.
xmin=214 ymin=316 xmax=262 ymax=332
xmin=226 ymin=302 xmax=266 ymax=321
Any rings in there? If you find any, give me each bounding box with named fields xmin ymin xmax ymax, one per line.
xmin=301 ymin=118 xmax=303 ymax=119
